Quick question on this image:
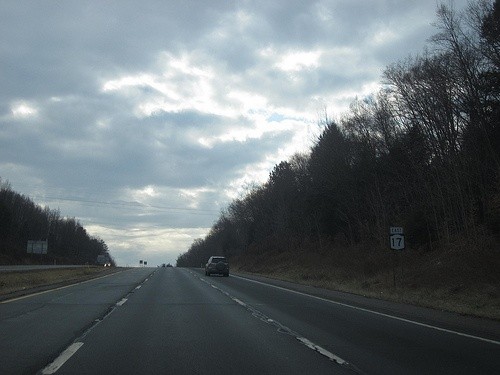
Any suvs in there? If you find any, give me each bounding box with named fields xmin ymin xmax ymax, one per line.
xmin=205 ymin=255 xmax=230 ymax=277
